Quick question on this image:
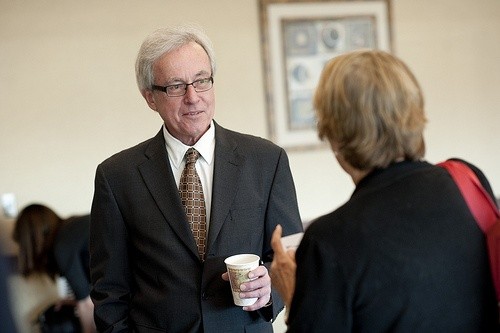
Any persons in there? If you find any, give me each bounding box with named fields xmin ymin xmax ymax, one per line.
xmin=90 ymin=29 xmax=305 ymax=332
xmin=11 ymin=203 xmax=98 ymax=333
xmin=269 ymin=50 xmax=500 ymax=333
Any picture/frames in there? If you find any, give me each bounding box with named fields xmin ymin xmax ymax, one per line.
xmin=258 ymin=0 xmax=393 ymax=149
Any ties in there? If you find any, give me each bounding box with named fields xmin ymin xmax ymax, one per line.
xmin=178 ymin=147 xmax=207 ymax=265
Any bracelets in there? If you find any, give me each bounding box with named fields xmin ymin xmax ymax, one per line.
xmin=263 ymin=295 xmax=273 ymax=307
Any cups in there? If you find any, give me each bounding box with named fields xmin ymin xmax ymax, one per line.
xmin=224 ymin=254 xmax=260 ymax=306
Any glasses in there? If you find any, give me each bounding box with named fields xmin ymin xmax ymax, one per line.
xmin=152 ymin=74 xmax=214 ymax=97
